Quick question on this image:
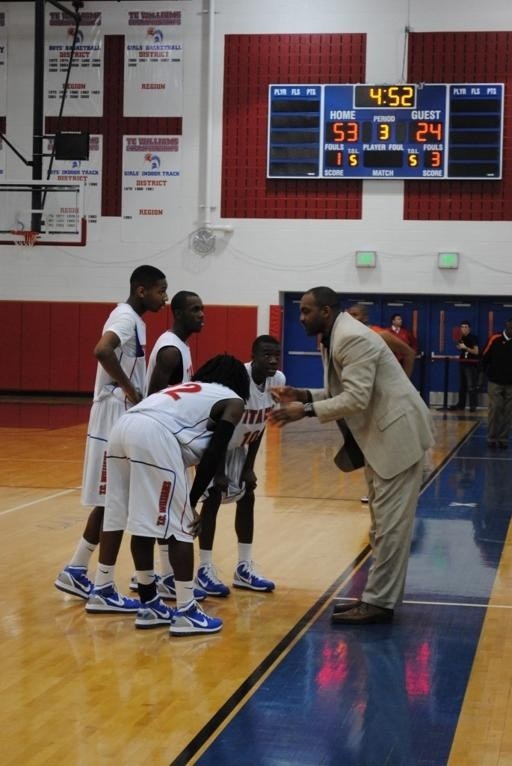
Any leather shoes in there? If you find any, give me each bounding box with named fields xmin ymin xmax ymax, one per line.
xmin=489 ymin=441 xmax=495 ymax=447
xmin=470 ymin=408 xmax=475 ymax=412
xmin=334 ymin=600 xmax=361 ymax=612
xmin=451 ymin=403 xmax=464 ymax=409
xmin=332 ymin=602 xmax=394 ymax=626
xmin=500 ymin=441 xmax=507 ymax=448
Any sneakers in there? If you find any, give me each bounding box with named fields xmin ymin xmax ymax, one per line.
xmin=134 ymin=597 xmax=178 ymax=629
xmin=130 ymin=571 xmax=177 ymax=600
xmin=195 ymin=564 xmax=230 ymax=597
xmin=233 ymin=561 xmax=275 ymax=591
xmin=54 ymin=567 xmax=95 ymax=599
xmin=168 ymin=599 xmax=223 ymax=637
xmin=85 ymin=581 xmax=141 ymax=613
xmin=164 ymin=573 xmax=207 ymax=601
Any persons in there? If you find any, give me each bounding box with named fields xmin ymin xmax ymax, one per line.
xmin=449 ymin=320 xmax=479 ymax=412
xmin=193 ymin=335 xmax=286 ymax=596
xmin=385 ymin=314 xmax=418 ymax=366
xmin=54 ymin=265 xmax=169 ymax=613
xmin=265 ymin=286 xmax=437 ymax=625
xmin=480 ymin=317 xmax=512 ymax=449
xmin=346 ymin=304 xmax=417 ymax=502
xmin=101 ymin=354 xmax=251 ymax=637
xmin=142 ymin=290 xmax=206 ymax=601
